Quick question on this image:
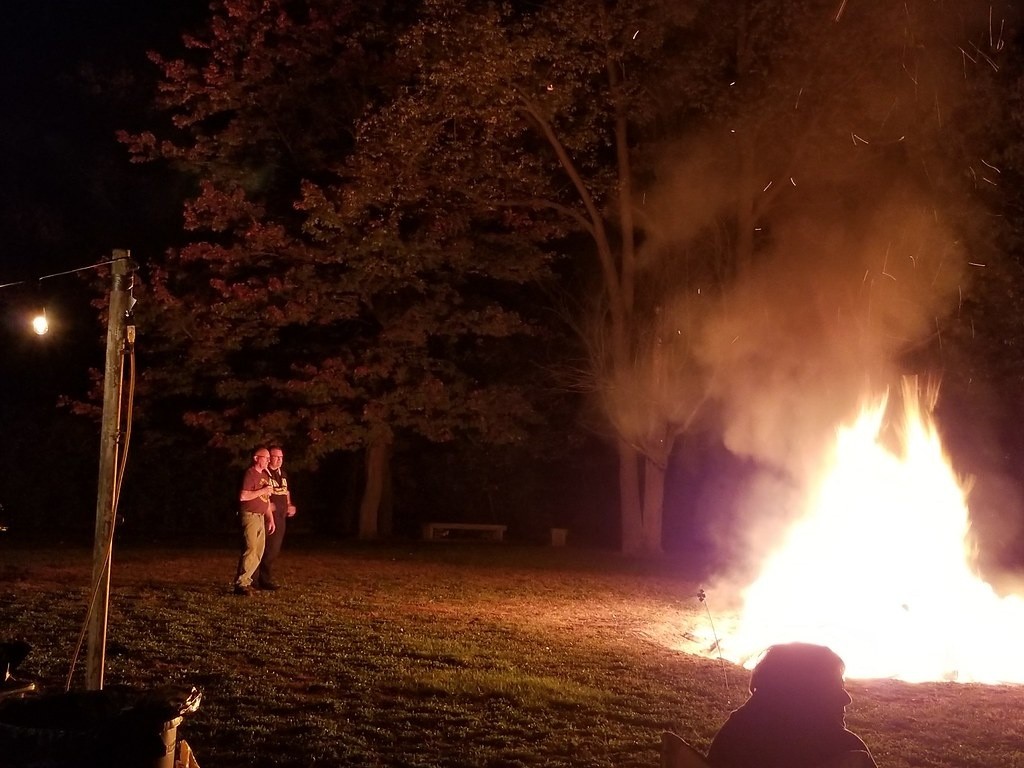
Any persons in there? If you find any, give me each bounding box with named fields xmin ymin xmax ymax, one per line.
xmin=256 ymin=446 xmax=296 ymax=590
xmin=233 ymin=447 xmax=276 ymax=595
xmin=705 ymin=641 xmax=879 ymax=768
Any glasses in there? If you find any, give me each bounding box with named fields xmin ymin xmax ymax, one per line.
xmin=258 ymin=456 xmax=270 ymax=460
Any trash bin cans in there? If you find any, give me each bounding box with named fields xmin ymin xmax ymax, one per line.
xmin=0 ymin=684 xmax=178 ymax=768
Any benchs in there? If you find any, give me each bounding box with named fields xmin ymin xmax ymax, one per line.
xmin=420 ymin=523 xmax=508 ymax=542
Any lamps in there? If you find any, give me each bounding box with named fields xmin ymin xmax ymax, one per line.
xmin=29 ymin=278 xmax=47 ymax=335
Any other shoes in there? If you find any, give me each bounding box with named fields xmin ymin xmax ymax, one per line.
xmin=235 ymin=587 xmax=252 ymax=595
xmin=259 ymin=579 xmax=279 ymax=590
